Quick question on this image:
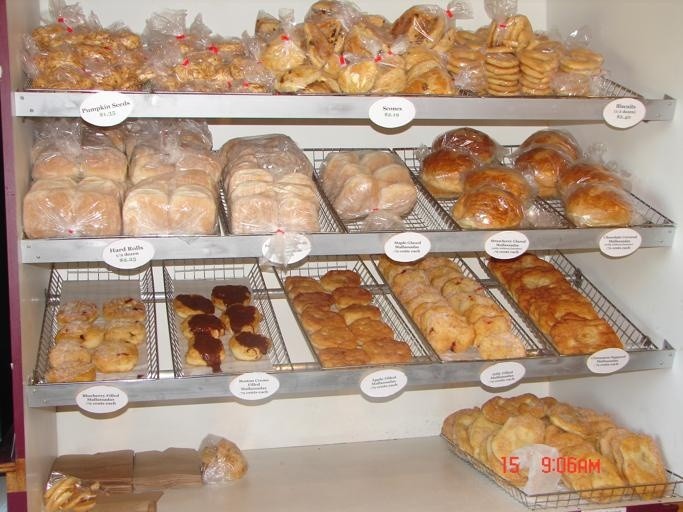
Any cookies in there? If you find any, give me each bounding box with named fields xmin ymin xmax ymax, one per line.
xmin=454 ymin=11 xmax=601 ymax=100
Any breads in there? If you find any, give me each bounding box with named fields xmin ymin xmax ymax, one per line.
xmin=434 ymin=127 xmax=493 ymax=160
xmin=518 ymin=149 xmax=570 ymax=196
xmin=43 ymin=291 xmax=152 ymax=384
xmin=378 ymin=257 xmax=526 ymax=358
xmin=321 ymin=147 xmax=415 ymax=226
xmin=185 ymin=337 xmax=225 ymax=367
xmin=569 ymin=187 xmax=628 ymax=225
xmin=29 ymin=21 xmax=262 ymax=92
xmin=172 ymin=292 xmax=215 ymax=317
xmin=211 ymin=284 xmax=254 ymax=307
xmin=219 ymin=308 xmax=263 ymax=333
xmin=218 ymin=134 xmax=322 ymax=232
xmin=23 ymin=125 xmax=124 ymax=239
xmin=521 ymin=129 xmax=577 ymax=161
xmin=454 ymin=192 xmax=521 ymax=229
xmin=441 ymin=395 xmax=668 ymax=499
xmin=485 ymin=255 xmax=623 ymax=355
xmin=180 ymin=314 xmax=223 ymax=335
xmin=460 ymin=166 xmax=530 ymax=203
xmin=281 ymin=272 xmax=410 ymax=368
xmin=424 ymin=151 xmax=463 ymax=201
xmin=229 ymin=333 xmax=270 ymax=362
xmin=256 ymin=4 xmax=453 ymax=93
xmin=557 ymin=164 xmax=617 ymax=200
xmin=206 ymin=436 xmax=244 ymax=481
xmin=120 ymin=119 xmax=219 ymax=236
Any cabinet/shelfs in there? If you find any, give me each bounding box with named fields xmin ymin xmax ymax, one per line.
xmin=12 ymin=75 xmax=681 ymax=512
